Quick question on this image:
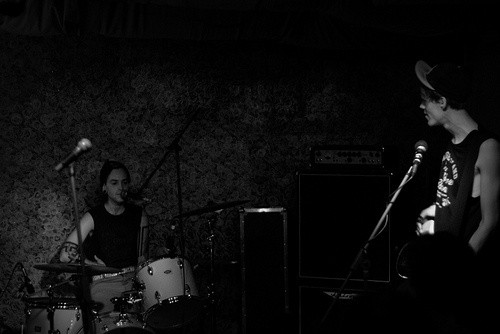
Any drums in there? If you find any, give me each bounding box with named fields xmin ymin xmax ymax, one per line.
xmin=20 ymin=294 xmax=85 ymax=334
xmin=86 ymin=266 xmax=146 ymax=334
xmin=134 ymin=254 xmax=203 ymax=328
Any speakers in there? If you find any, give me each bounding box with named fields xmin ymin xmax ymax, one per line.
xmin=298 ymin=173 xmax=392 ymax=283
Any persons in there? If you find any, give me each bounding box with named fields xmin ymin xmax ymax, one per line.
xmin=411 ymin=55 xmax=500 ymax=256
xmin=60 ymin=161 xmax=149 ymax=266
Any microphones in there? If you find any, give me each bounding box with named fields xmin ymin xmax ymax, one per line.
xmin=54 ymin=138 xmax=92 ymax=171
xmin=412 ymin=141 xmax=427 ymax=166
xmin=20 ymin=262 xmax=35 ymax=294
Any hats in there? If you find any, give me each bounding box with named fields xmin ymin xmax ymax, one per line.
xmin=415 ymin=58 xmax=472 ymax=103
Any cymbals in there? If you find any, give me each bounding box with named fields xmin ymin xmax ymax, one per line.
xmin=173 ymin=198 xmax=253 ymax=219
xmin=33 ymin=262 xmax=123 ymax=274
